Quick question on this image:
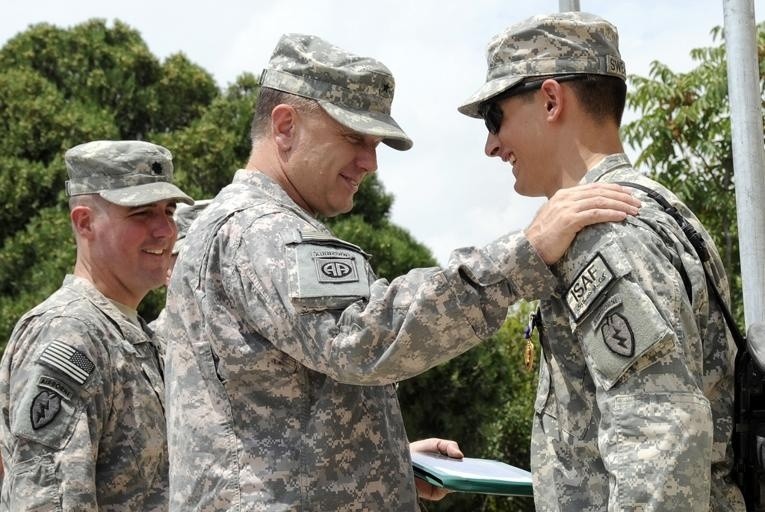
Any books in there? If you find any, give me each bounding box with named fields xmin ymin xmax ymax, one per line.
xmin=410 ymin=450 xmax=535 ymax=498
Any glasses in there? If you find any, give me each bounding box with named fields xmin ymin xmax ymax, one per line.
xmin=477 ymin=74 xmax=581 ymax=135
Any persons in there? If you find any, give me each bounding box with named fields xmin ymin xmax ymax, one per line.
xmin=1 ymin=139 xmax=195 ymax=512
xmin=147 ymin=197 xmax=214 ymax=371
xmin=161 ymin=32 xmax=642 ymax=512
xmin=456 ymin=11 xmax=747 ymax=512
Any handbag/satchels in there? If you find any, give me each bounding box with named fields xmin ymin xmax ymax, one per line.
xmin=732 ymin=343 xmax=764 ymax=511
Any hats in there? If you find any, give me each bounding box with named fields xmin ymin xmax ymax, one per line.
xmin=458 ymin=11 xmax=626 ymax=118
xmin=257 ymin=33 xmax=412 ymax=151
xmin=65 ymin=141 xmax=194 ymax=207
xmin=172 ymin=199 xmax=213 ymax=254
